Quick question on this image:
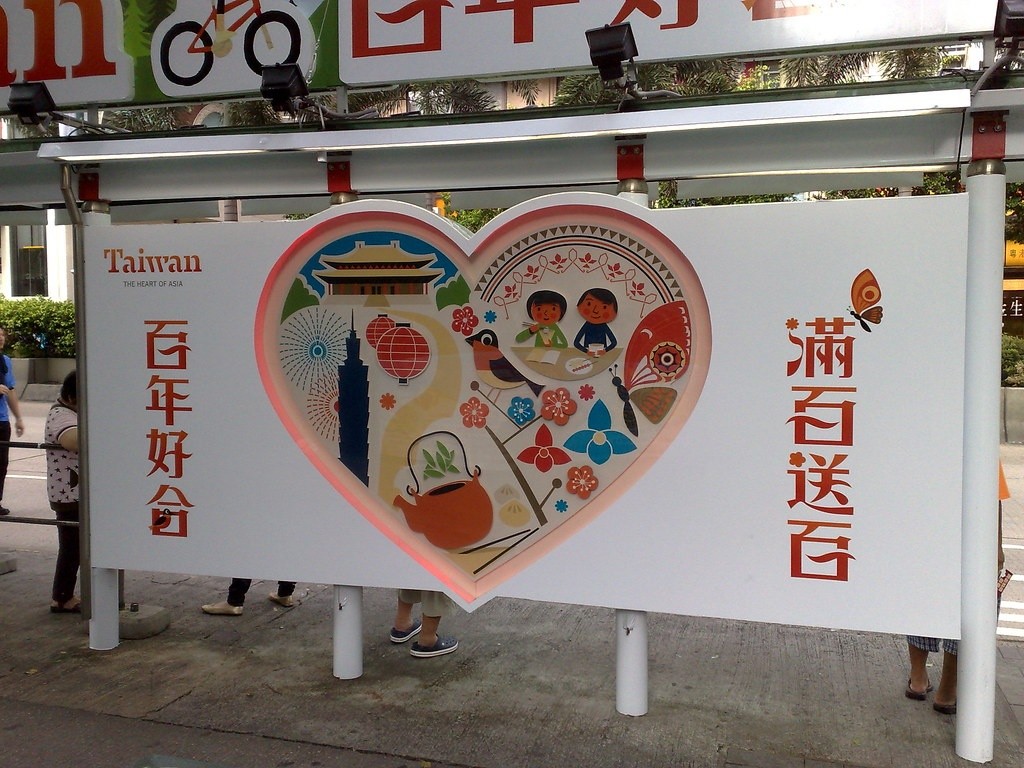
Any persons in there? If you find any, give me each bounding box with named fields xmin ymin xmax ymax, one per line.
xmin=905 ymin=462 xmax=1010 ymax=712
xmin=388 ymin=589 xmax=459 ymax=658
xmin=200 ymin=579 xmax=298 ymax=616
xmin=0 ymin=327 xmax=24 ymax=516
xmin=43 ymin=369 xmax=83 ymax=614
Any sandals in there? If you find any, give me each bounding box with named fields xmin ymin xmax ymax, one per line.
xmin=408 ymin=636 xmax=459 ymax=657
xmin=389 ymin=618 xmax=423 ymax=644
xmin=50 ymin=597 xmax=83 ymax=615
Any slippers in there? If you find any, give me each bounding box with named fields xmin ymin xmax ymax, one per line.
xmin=905 ymin=676 xmax=933 ymax=701
xmin=932 ymin=698 xmax=957 ymax=715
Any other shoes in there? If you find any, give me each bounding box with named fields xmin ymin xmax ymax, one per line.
xmin=0 ymin=507 xmax=11 ymax=515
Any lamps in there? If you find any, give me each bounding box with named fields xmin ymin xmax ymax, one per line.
xmin=585 ymin=22 xmax=683 ymax=117
xmin=8 ymin=81 xmax=131 ymax=137
xmin=261 ymin=62 xmax=380 ymax=130
xmin=939 ymin=0 xmax=1024 ymax=97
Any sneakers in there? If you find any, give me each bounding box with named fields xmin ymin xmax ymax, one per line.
xmin=270 ymin=590 xmax=293 ymax=607
xmin=201 ymin=600 xmax=244 ymax=615
